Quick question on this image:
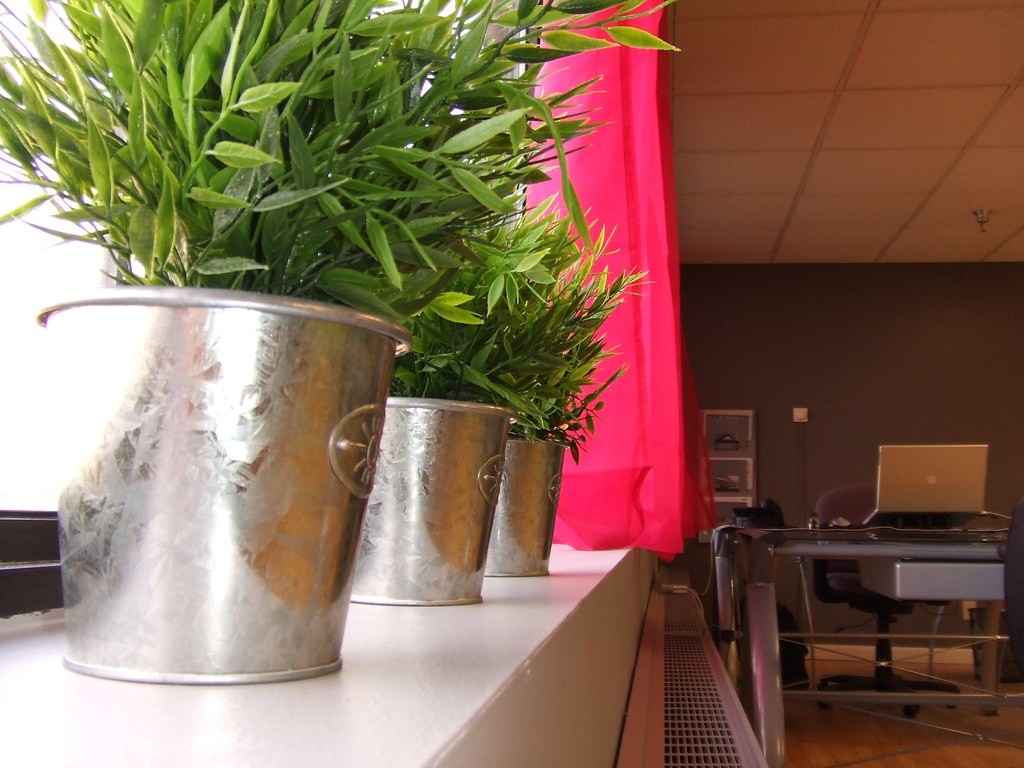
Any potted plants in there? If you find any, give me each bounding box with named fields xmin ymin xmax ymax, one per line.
xmin=0 ymin=0 xmax=684 ymax=686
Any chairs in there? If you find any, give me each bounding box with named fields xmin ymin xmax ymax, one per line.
xmin=811 ymin=486 xmax=961 ymax=718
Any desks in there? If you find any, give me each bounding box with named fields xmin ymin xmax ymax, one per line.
xmin=714 ymin=526 xmax=1007 ymax=714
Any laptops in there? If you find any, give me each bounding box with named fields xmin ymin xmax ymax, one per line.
xmin=863 ymin=445 xmax=989 ymax=525
